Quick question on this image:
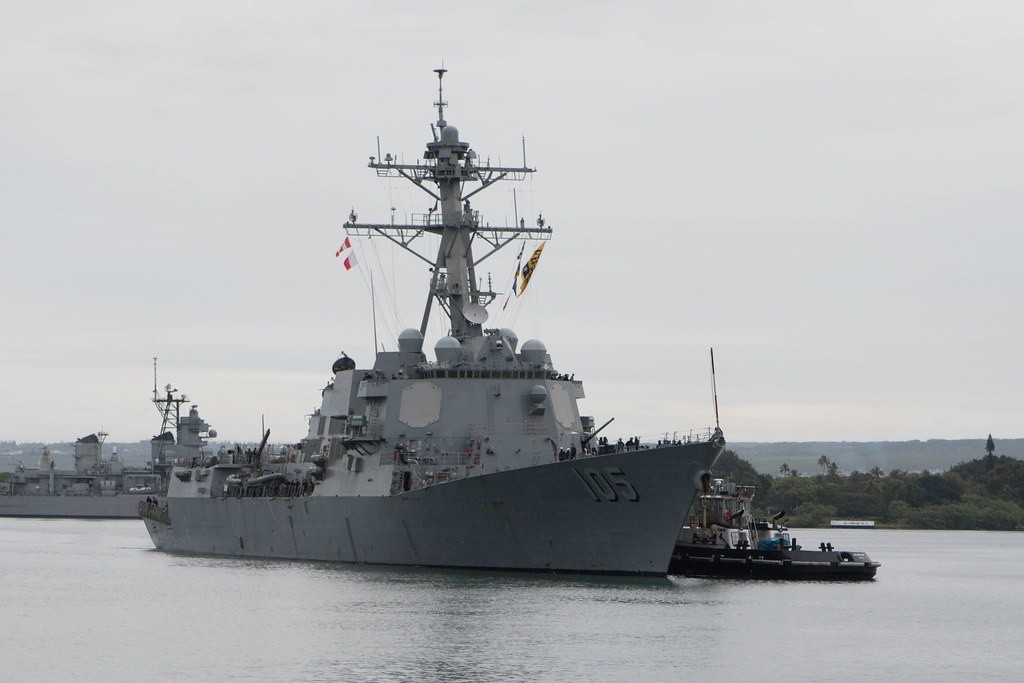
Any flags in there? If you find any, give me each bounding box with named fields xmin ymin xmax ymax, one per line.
xmin=343 ymin=251 xmax=358 ymax=270
xmin=502 ymin=241 xmax=547 ymax=312
xmin=336 ymin=237 xmax=351 ymax=257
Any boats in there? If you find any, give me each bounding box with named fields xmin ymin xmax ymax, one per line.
xmin=671 ymin=478 xmax=881 ymax=578
xmin=0 ymin=430 xmax=175 ymax=517
xmin=138 ymin=62 xmax=725 ymax=574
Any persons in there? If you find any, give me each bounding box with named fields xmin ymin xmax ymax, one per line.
xmin=363 ymin=372 xmax=372 ymax=380
xmin=558 ymin=444 xmax=576 ymax=461
xmin=152 ymin=497 xmax=158 ymax=505
xmin=616 ymin=437 xmax=639 ymax=453
xmin=596 ymin=437 xmax=608 ymax=454
xmin=392 ymin=374 xmax=396 ymax=379
xmin=248 ymin=449 xmax=252 ymax=463
xmin=557 ymin=373 xmax=575 ymax=381
xmin=147 ymin=496 xmax=151 ymax=503
xmin=253 ymin=448 xmax=257 ymax=453
